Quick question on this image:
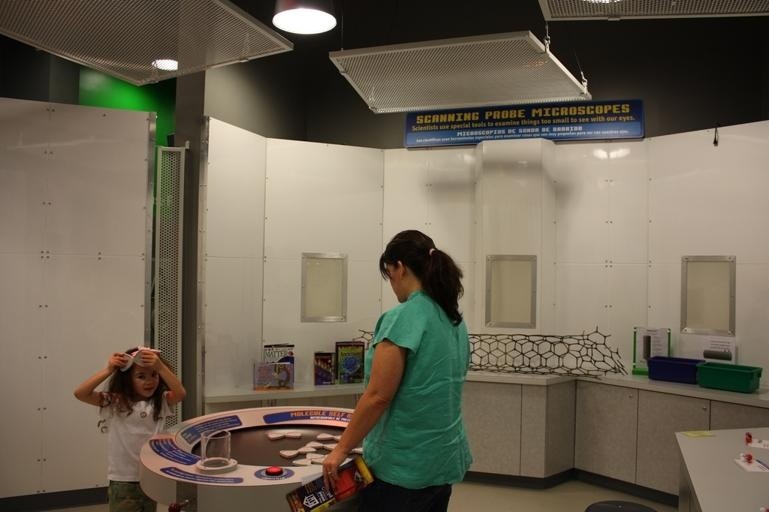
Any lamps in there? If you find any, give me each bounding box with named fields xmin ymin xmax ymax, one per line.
xmin=270 ymin=1 xmax=338 ymax=35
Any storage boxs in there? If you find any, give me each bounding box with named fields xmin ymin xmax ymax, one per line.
xmin=646 ymin=354 xmax=762 ymax=393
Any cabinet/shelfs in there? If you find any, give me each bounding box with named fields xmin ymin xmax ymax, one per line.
xmin=572 ymin=381 xmax=636 ymax=484
xmin=459 ymin=382 xmax=521 ymax=476
xmin=637 ymin=388 xmax=710 ymax=493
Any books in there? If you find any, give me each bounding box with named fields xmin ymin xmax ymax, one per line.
xmin=262 ymin=342 xmax=295 ymax=361
xmin=251 ymin=359 xmax=295 ymax=391
xmin=313 ymin=349 xmax=337 ymax=388
xmin=335 ymin=340 xmax=365 ymax=384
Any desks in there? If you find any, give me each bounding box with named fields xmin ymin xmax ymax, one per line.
xmin=677 ymin=429 xmax=769 ymax=511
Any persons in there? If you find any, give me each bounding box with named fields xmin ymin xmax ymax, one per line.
xmin=278 ymin=350 xmax=295 ymax=362
xmin=264 ymin=365 xmax=288 ymax=388
xmin=73 ymin=345 xmax=186 ymax=512
xmin=321 ymin=229 xmax=473 ymax=511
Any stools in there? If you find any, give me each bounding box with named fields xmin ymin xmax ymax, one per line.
xmin=586 ymin=501 xmax=657 ymax=512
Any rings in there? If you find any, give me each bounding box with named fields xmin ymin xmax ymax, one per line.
xmin=327 ymin=471 xmax=331 ymax=477
